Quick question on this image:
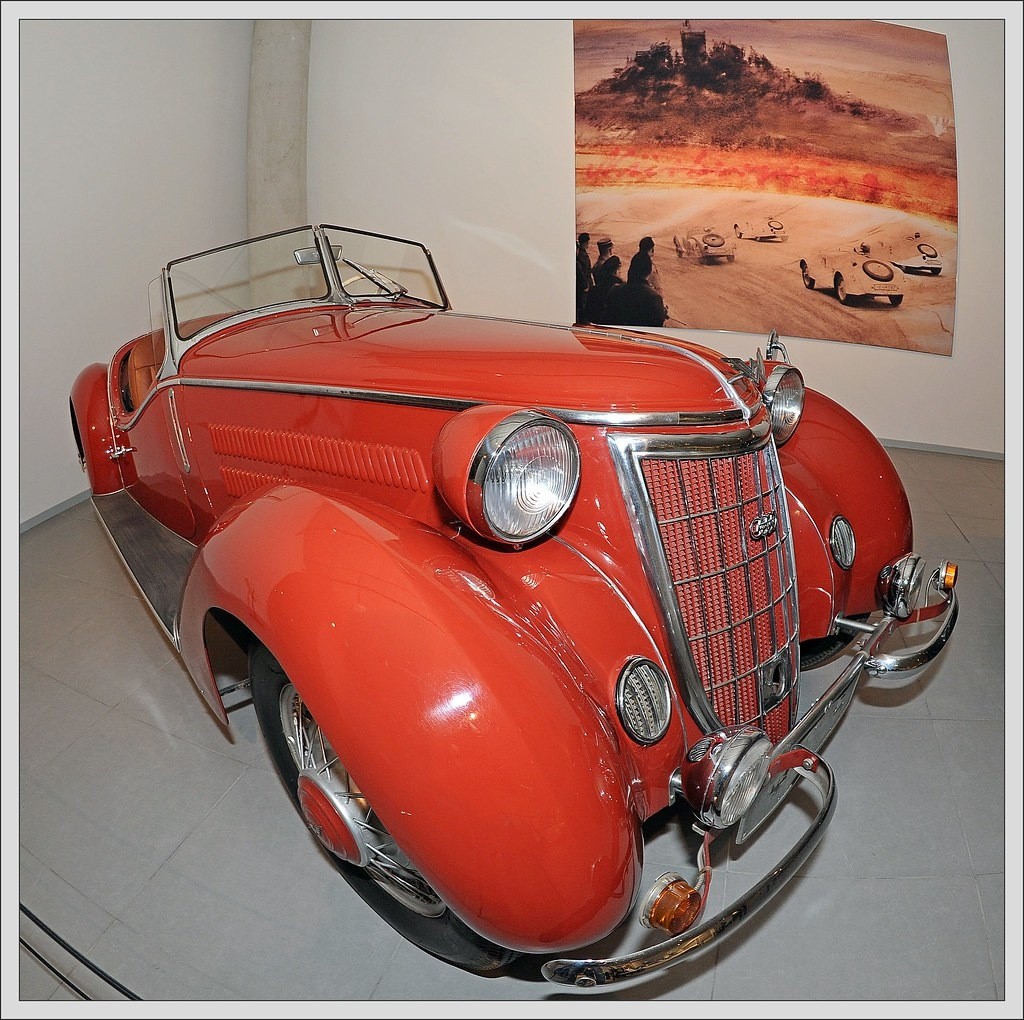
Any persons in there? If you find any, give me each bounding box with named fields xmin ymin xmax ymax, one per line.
xmin=576 ymin=233 xmax=669 ymax=327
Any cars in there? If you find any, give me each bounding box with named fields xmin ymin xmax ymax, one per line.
xmin=798 ymin=241 xmax=908 ymax=305
xmin=62 ymin=226 xmax=963 ymax=990
xmin=734 ymin=217 xmax=788 ymax=242
xmin=671 ymin=226 xmax=737 ymax=262
xmin=888 ymin=242 xmax=943 ymax=276
